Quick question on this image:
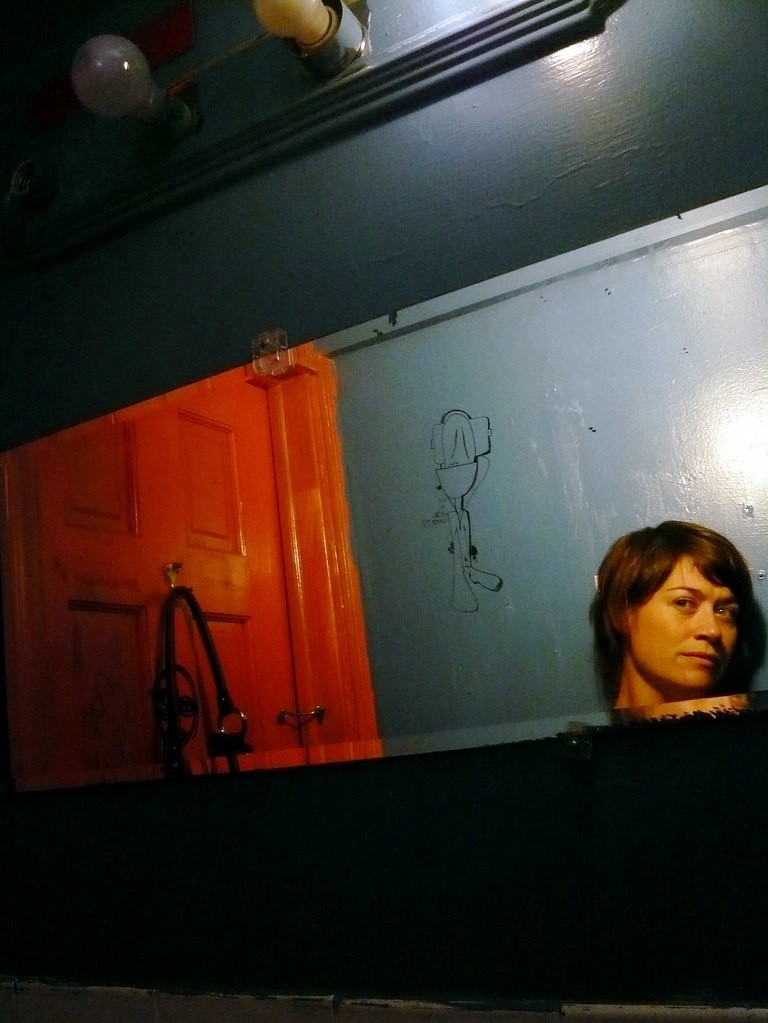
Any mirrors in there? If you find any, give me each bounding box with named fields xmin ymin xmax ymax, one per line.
xmin=0 ymin=187 xmax=768 ymax=792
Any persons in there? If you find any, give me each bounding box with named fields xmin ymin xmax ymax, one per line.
xmin=591 ymin=519 xmax=756 ymax=722
